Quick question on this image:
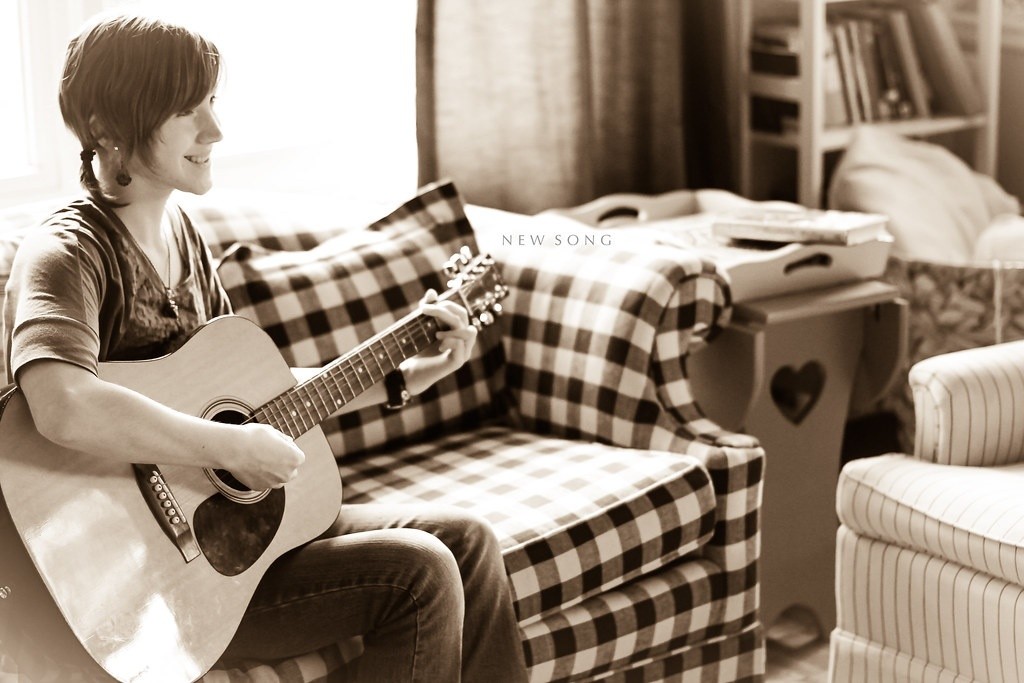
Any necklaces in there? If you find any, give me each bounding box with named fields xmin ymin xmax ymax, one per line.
xmin=130 ymin=225 xmax=178 ymax=318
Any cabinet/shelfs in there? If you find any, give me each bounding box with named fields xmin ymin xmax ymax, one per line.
xmin=728 ymin=0 xmax=1003 ymax=226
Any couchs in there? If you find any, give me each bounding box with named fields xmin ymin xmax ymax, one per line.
xmin=1 ymin=206 xmax=769 ymax=683
xmin=827 ymin=343 xmax=1023 ymax=681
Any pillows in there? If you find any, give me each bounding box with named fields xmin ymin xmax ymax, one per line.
xmin=210 ymin=175 xmax=514 ymax=461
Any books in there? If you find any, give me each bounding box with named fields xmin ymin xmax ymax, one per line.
xmin=710 ymin=200 xmax=888 ymax=246
xmin=750 ymin=0 xmax=982 ymax=131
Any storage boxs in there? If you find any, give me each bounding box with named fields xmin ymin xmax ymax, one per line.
xmin=532 ymin=187 xmax=897 ymax=301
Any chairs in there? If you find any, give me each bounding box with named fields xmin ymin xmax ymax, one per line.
xmin=829 ymin=125 xmax=1023 ymax=451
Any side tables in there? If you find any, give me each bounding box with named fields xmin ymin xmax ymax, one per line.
xmin=692 ymin=276 xmax=913 ymax=645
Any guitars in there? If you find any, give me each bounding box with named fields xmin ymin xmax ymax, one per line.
xmin=0 ymin=244 xmax=510 ymax=683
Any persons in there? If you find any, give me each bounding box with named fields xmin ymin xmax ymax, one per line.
xmin=6 ymin=17 xmax=530 ymax=683
